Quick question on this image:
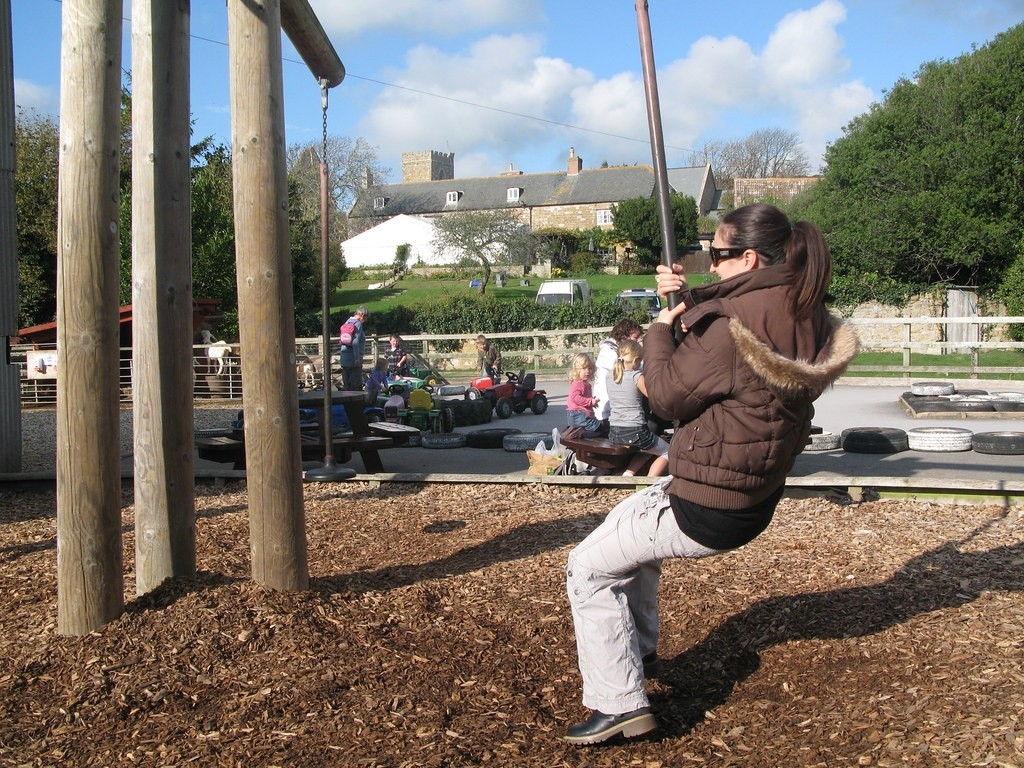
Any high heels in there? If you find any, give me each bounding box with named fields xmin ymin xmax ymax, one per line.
xmin=641 ymin=650 xmax=664 ymax=676
xmin=564 ymin=705 xmax=657 ymax=746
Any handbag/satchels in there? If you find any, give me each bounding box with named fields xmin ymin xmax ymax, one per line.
xmin=526 ymin=428 xmax=595 ymax=476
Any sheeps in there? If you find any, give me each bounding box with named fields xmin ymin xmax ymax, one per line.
xmin=200 ymin=329 xmax=233 ymax=376
xmin=296 ymin=356 xmax=316 ymax=389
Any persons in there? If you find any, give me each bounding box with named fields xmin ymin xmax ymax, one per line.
xmin=339 ymin=307 xmax=370 ymax=391
xmin=590 ymin=318 xmax=645 ymax=437
xmin=563 ymin=353 xmax=606 ymax=439
xmin=562 ymin=202 xmax=858 ymax=745
xmin=382 ymin=333 xmax=407 ymax=377
xmin=475 ymin=335 xmax=502 ymax=384
xmin=606 ymin=339 xmax=671 ymax=477
xmin=365 ymin=359 xmax=390 ymax=406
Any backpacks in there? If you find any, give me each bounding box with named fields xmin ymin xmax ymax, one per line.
xmin=339 ymin=319 xmax=359 ymax=346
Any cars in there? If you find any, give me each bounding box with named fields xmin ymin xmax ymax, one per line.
xmin=615 ymin=289 xmax=661 ymax=318
xmin=296 ymin=355 xmax=316 ymax=373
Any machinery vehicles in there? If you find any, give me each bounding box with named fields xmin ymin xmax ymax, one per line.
xmin=373 ymin=384 xmax=409 ymax=425
xmin=465 ymin=366 xmax=504 ymax=400
xmin=482 ymin=368 xmax=548 ymax=419
xmin=386 ymin=363 xmax=428 ymax=389
xmin=397 ymin=385 xmax=455 ymax=434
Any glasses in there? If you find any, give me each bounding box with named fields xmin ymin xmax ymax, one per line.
xmin=709 ymin=246 xmax=774 ymax=266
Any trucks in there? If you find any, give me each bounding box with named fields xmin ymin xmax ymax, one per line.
xmin=535 ymin=279 xmax=589 ymax=306
